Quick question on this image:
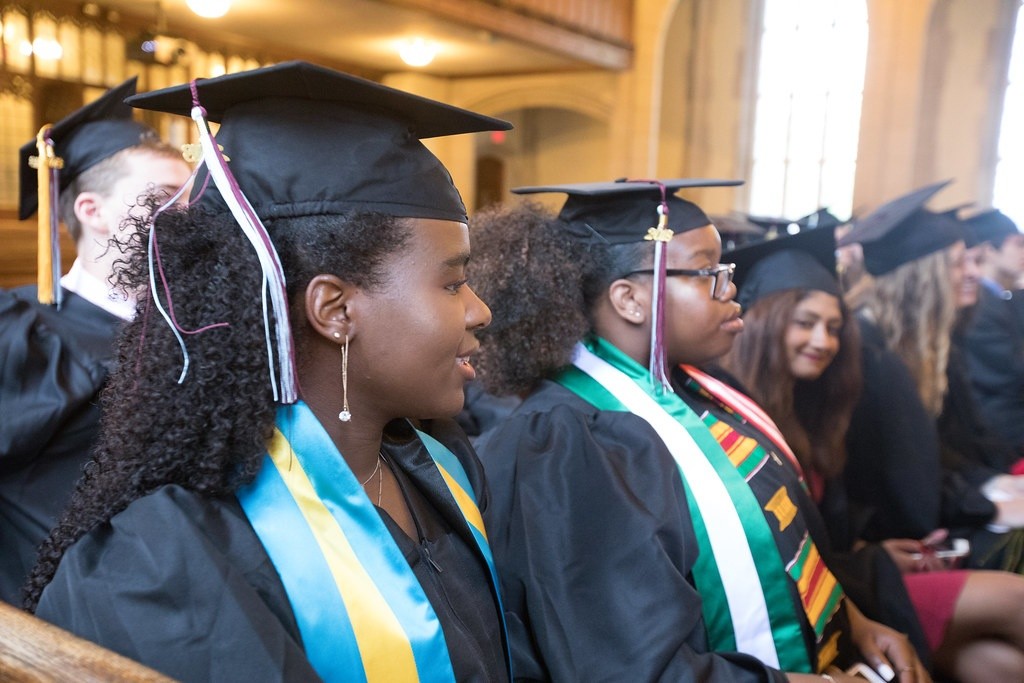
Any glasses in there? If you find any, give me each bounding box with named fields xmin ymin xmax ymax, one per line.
xmin=611 ymin=264 xmax=739 ymax=299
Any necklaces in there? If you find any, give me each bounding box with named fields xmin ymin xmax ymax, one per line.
xmin=362 ymin=455 xmax=383 ymax=507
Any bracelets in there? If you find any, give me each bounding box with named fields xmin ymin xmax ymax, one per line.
xmin=821 ymin=674 xmax=836 ymax=683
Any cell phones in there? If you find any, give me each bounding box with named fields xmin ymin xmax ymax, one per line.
xmin=913 ymin=538 xmax=970 ymax=560
xmin=847 ymin=663 xmax=887 ymax=683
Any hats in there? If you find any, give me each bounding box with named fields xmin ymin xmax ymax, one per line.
xmin=723 ymin=223 xmax=845 ymax=312
xmin=18 ymin=75 xmax=160 ymax=304
xmin=120 ymin=62 xmax=516 ymax=403
xmin=508 ymin=176 xmax=746 ymax=395
xmin=836 ymin=178 xmax=1016 ymax=277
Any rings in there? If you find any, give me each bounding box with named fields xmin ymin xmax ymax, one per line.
xmin=899 ymin=665 xmax=914 ymax=672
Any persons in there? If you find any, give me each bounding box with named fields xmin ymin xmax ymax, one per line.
xmin=0 ymin=57 xmax=1024 ymax=683
